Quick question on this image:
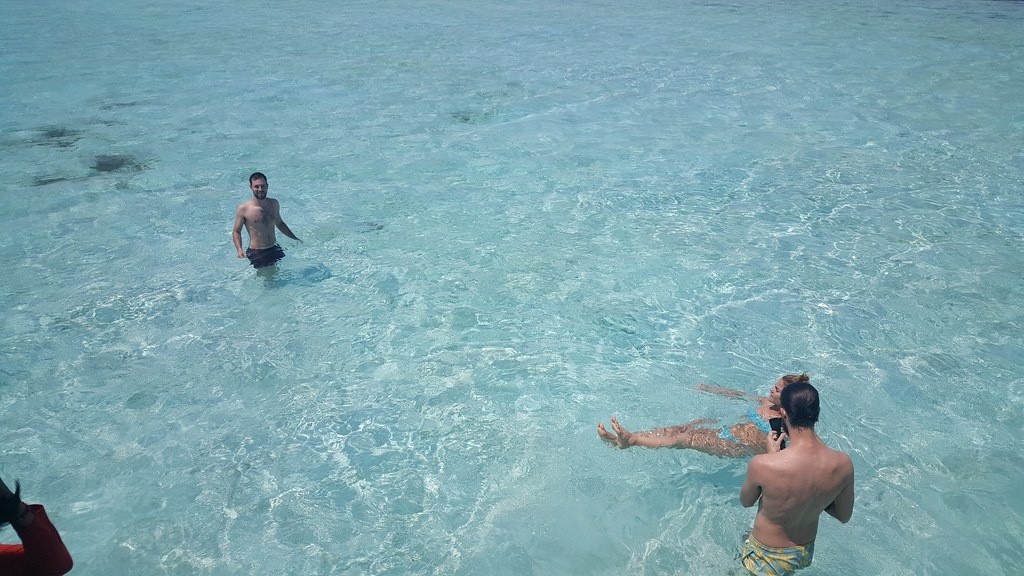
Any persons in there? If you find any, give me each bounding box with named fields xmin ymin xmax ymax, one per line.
xmin=739 ymin=382 xmax=854 ymax=576
xmin=596 ymin=371 xmax=809 ymax=459
xmin=0 ymin=476 xmax=74 ymax=576
xmin=233 ymin=172 xmax=303 ymax=267
